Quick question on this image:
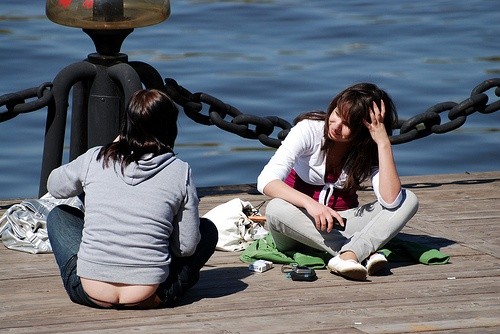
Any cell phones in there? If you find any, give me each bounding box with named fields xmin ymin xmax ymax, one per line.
xmin=326 ymin=217 xmax=347 ymax=231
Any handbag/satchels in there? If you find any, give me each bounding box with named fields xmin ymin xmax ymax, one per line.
xmin=0 ymin=191 xmax=88 ymax=254
xmin=200 ymin=198 xmax=270 ymax=252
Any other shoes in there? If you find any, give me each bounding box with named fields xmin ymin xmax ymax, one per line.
xmin=365 ymin=251 xmax=388 ymax=275
xmin=327 ymin=253 xmax=368 ymax=280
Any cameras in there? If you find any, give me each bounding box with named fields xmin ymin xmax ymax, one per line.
xmin=293 ymin=266 xmax=310 ymax=273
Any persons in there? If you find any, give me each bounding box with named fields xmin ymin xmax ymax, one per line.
xmin=46 ymin=88 xmax=220 ymax=313
xmin=256 ymin=81 xmax=420 ymax=282
